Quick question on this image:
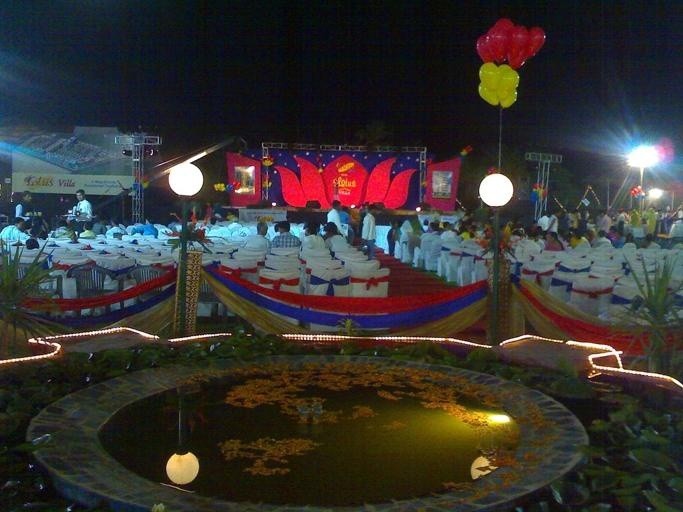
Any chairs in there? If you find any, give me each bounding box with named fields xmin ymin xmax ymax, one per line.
xmin=375 ymin=220 xmax=682 ymax=329
xmin=2 ymin=219 xmax=392 ymax=329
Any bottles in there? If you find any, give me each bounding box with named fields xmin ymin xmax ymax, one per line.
xmin=72 ymin=202 xmax=80 ymax=215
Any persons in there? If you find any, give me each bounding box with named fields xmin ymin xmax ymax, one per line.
xmin=15 ymin=190 xmax=33 ymax=228
xmin=529 ymin=205 xmax=683 ymax=251
xmin=327 ymin=201 xmax=391 ymax=257
xmin=241 ymin=221 xmax=347 ymax=249
xmin=391 ymin=205 xmax=525 ymax=258
xmin=161 ymin=200 xmax=238 ymax=222
xmin=66 ymin=189 xmax=92 ymax=233
xmin=0 ymin=215 xmax=160 ymax=250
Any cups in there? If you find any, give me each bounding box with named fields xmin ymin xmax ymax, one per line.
xmin=23 ymin=211 xmax=43 ymax=216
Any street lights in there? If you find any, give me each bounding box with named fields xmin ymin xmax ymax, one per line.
xmin=168 ymin=162 xmax=204 ymax=337
xmin=479 ymin=172 xmax=514 ymax=347
xmin=165 ymin=389 xmax=200 ymax=487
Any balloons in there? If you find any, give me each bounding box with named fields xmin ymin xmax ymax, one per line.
xmin=530 ymin=183 xmax=548 ymax=204
xmin=213 ymin=181 xmax=241 ymax=192
xmin=460 ymin=144 xmax=473 ymax=157
xmin=127 ymin=173 xmax=150 ymax=195
xmin=482 ymin=165 xmax=498 ymax=177
xmin=630 ymin=185 xmax=646 ymax=200
xmin=261 ymin=154 xmax=273 ymax=190
xmin=475 ymin=17 xmax=546 ymax=110
